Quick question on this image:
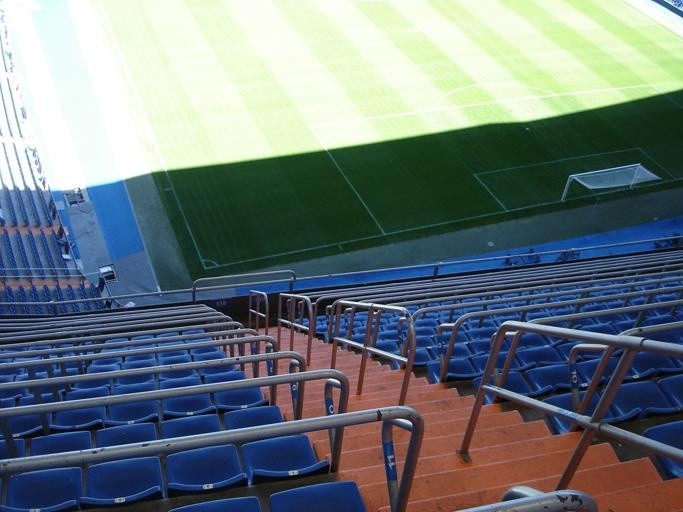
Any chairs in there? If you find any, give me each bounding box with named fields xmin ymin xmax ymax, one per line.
xmin=0 ymin=325 xmax=376 ymax=512
xmin=0 ymin=140 xmax=106 ymax=315
xmin=290 ymin=250 xmax=682 ymax=478
